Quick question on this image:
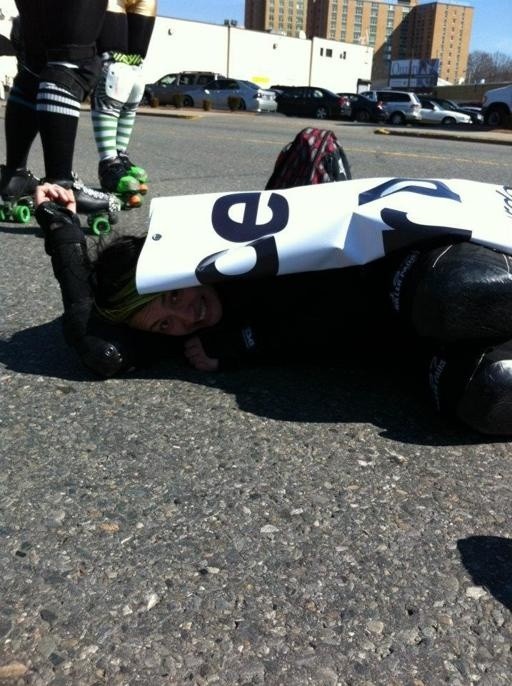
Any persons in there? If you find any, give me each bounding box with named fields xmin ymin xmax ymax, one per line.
xmin=91 ymin=1 xmax=156 ymax=210
xmin=35 ymin=180 xmax=511 ymax=440
xmin=1 ymin=0 xmax=123 ymax=236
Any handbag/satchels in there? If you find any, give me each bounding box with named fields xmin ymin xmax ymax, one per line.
xmin=265 ymin=127 xmax=351 ymax=190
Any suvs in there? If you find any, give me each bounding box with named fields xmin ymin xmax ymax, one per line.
xmin=144 ymin=70 xmax=224 ymax=107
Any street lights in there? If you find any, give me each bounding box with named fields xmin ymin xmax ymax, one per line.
xmin=225 ymin=17 xmax=237 ymax=77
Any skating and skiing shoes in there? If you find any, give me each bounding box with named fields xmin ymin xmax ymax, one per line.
xmin=0 ymin=164 xmax=43 ymax=223
xmin=98 ymin=156 xmax=144 ymax=207
xmin=37 ymin=171 xmax=122 ymax=234
xmin=117 ymin=149 xmax=148 ymax=195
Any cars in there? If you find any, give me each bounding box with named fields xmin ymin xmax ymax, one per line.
xmin=482 ymin=83 xmax=511 ymax=128
xmin=184 ymin=78 xmax=277 ymax=113
xmin=268 ymin=83 xmax=486 ymax=127
